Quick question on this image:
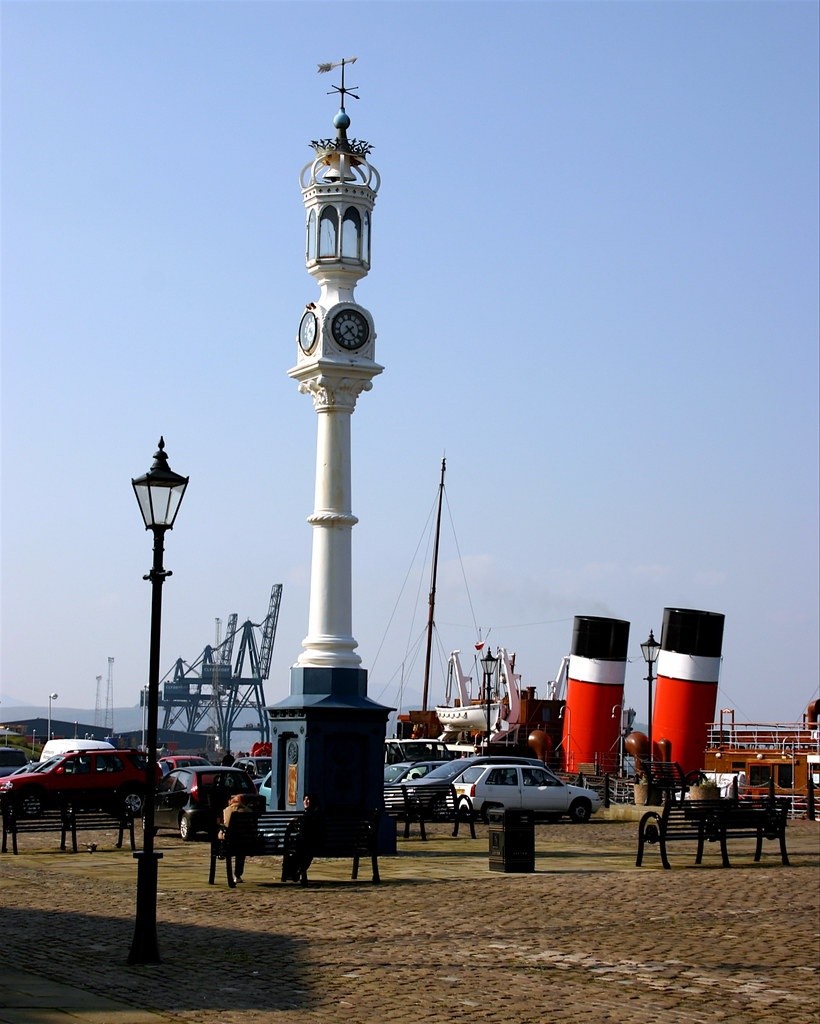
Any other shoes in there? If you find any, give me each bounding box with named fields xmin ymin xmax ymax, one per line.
xmin=232 ymin=876 xmax=243 ymax=881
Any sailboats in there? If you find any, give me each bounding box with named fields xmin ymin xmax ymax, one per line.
xmin=368 ymin=457 xmax=636 ymax=774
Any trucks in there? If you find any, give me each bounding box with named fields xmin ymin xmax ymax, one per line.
xmin=384 ymin=738 xmax=453 ymax=763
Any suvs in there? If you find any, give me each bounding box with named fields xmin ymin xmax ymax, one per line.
xmin=142 ymin=765 xmax=258 ymax=841
xmin=0 ymin=748 xmax=163 ymax=818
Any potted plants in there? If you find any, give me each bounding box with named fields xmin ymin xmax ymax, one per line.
xmin=689 ymin=778 xmax=721 ymax=807
xmin=635 ymin=775 xmax=663 ymax=806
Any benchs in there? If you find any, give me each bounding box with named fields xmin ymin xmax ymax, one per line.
xmin=383 ymin=784 xmax=476 ymax=840
xmin=640 ymin=760 xmax=708 ymax=808
xmin=0 ymin=789 xmax=135 ymax=855
xmin=636 ymin=797 xmax=793 ymax=869
xmin=208 ymin=808 xmax=383 ymax=888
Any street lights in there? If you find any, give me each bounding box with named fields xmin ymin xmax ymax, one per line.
xmin=480 ymin=646 xmax=498 ymax=756
xmin=89 ymin=737 xmax=90 ymax=740
xmin=6 ymin=728 xmax=10 ymax=746
xmin=782 ymin=737 xmax=795 ymax=820
xmin=612 ymin=705 xmax=623 ymax=778
xmin=127 ymin=434 xmax=189 ymax=966
xmin=558 ymin=706 xmax=571 ymax=772
xmin=52 ymin=732 xmax=54 ymax=740
xmin=48 ymin=693 xmax=58 ymax=740
xmin=74 ymin=720 xmax=78 ymax=739
xmin=91 ymin=734 xmax=94 ymax=739
xmin=640 ymin=629 xmax=662 ymax=783
xmin=474 ymin=733 xmax=484 ymax=756
xmin=85 ymin=733 xmax=88 ymax=739
xmin=32 ymin=730 xmax=36 ymax=754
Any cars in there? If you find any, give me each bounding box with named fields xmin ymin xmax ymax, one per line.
xmin=231 ymin=757 xmax=272 ymax=780
xmin=0 ymin=748 xmax=29 ymax=777
xmin=384 ymin=756 xmax=554 ymax=808
xmin=156 ymin=756 xmax=213 ymax=776
xmin=258 ymin=770 xmax=272 ymax=806
xmin=445 ymin=765 xmax=601 ymax=823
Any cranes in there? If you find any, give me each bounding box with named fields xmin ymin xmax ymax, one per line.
xmin=105 ymin=656 xmax=114 ymax=728
xmin=94 ymin=675 xmax=102 ymax=726
xmin=158 ymin=584 xmax=283 ymax=755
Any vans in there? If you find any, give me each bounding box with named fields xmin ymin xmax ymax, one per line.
xmin=40 ymin=739 xmax=116 ymax=772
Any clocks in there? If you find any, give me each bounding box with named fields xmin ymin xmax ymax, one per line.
xmin=332 ymin=310 xmax=369 ymax=350
xmin=299 ymin=312 xmax=317 ymax=351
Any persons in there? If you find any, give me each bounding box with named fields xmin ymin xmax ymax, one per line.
xmin=222 ymin=751 xmax=235 ymax=767
xmin=283 ymin=792 xmax=327 ymax=882
